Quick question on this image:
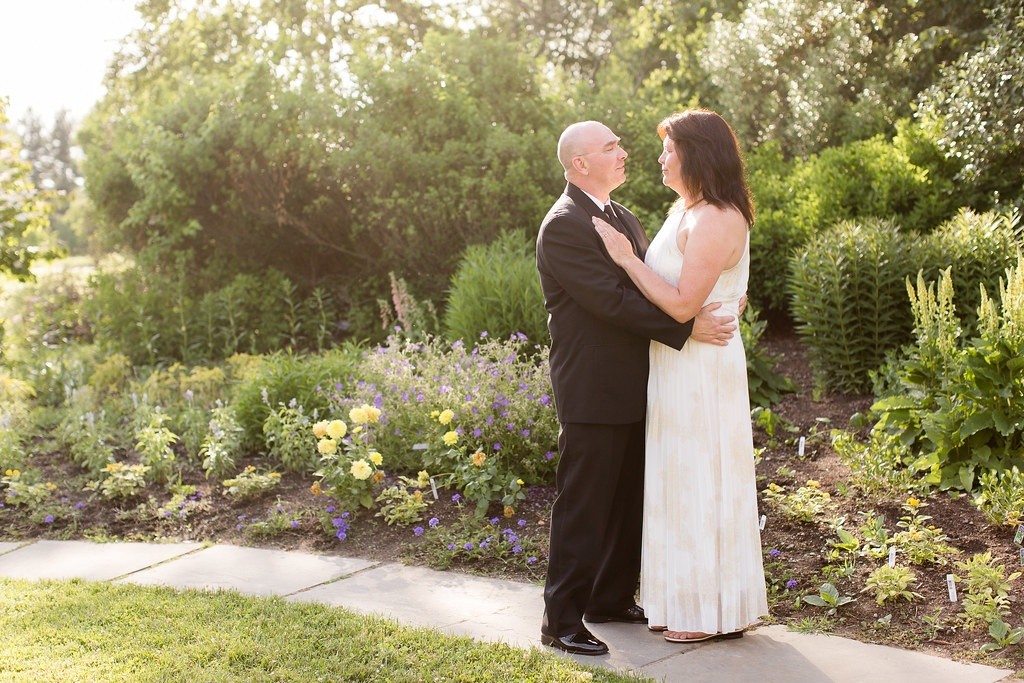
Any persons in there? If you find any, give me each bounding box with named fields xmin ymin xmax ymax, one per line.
xmin=534 ymin=120 xmax=749 ymax=658
xmin=591 ymin=108 xmax=770 ymax=642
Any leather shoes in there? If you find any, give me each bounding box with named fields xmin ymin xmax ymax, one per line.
xmin=541 ymin=628 xmax=608 ymax=655
xmin=584 ymin=604 xmax=649 ymax=624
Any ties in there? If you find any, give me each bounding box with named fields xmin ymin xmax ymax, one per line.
xmin=605 ymin=205 xmax=639 ymax=257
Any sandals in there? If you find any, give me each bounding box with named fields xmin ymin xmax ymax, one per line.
xmin=647 ymin=622 xmax=668 ymax=632
xmin=664 ymin=625 xmax=743 ymax=643
